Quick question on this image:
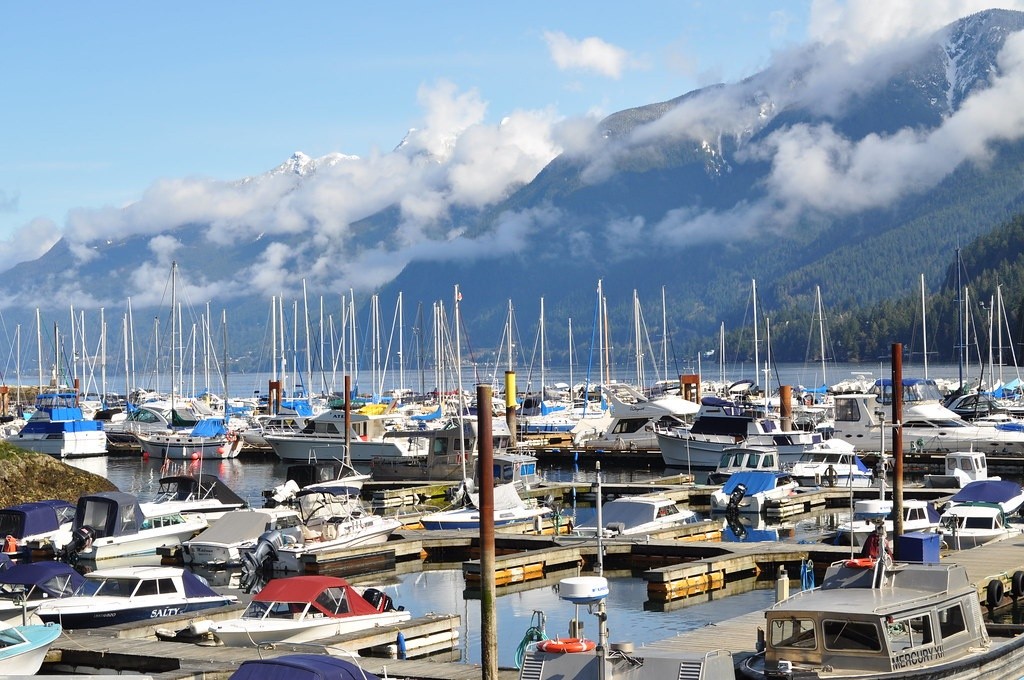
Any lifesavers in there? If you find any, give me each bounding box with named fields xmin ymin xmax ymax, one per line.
xmin=226 ymin=430 xmax=237 ymax=442
xmin=987 ymin=579 xmax=1005 ymax=607
xmin=1011 ymin=571 xmax=1024 ymax=597
xmin=844 ymin=558 xmax=878 ymax=568
xmin=537 ymin=638 xmax=595 ymax=653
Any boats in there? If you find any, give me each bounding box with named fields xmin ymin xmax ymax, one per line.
xmin=711 ymin=472 xmax=799 ymax=513
xmin=790 ymin=449 xmax=874 ymax=487
xmin=707 ymin=445 xmax=779 ymax=485
xmin=22 ymin=491 xmax=209 ymax=576
xmin=936 ymin=503 xmax=1020 ymax=550
xmin=924 ymin=451 xmax=1001 ymax=490
xmin=1 ymin=499 xmax=78 ymax=565
xmin=739 ymin=516 xmax=1024 ymax=680
xmin=0 ymin=622 xmax=62 ymax=675
xmin=836 ymin=412 xmax=943 ymax=546
xmin=0 ymin=554 xmax=100 ymax=621
xmin=209 ymin=576 xmax=411 ymax=647
xmin=182 ymin=486 xmax=403 ymax=587
xmin=34 ymin=567 xmax=242 ymax=629
xmin=572 ymin=496 xmax=696 ymax=537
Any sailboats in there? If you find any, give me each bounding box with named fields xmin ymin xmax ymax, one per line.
xmin=420 ymin=285 xmax=565 ymax=531
xmin=1 ymin=247 xmax=1024 ymax=482
